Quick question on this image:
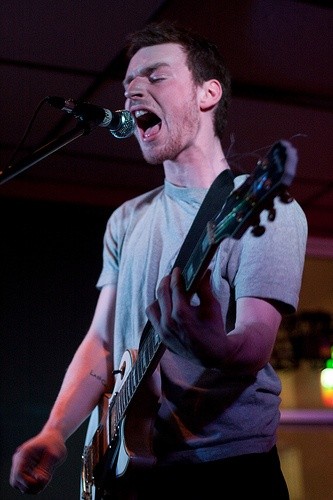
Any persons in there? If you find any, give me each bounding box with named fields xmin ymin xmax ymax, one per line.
xmin=6 ymin=19 xmax=312 ymax=500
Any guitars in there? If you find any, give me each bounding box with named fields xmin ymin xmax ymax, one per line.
xmin=80 ymin=133 xmax=298 ymax=500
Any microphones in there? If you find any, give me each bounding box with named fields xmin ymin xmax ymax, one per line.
xmin=45 ymin=96 xmax=137 ymax=139
